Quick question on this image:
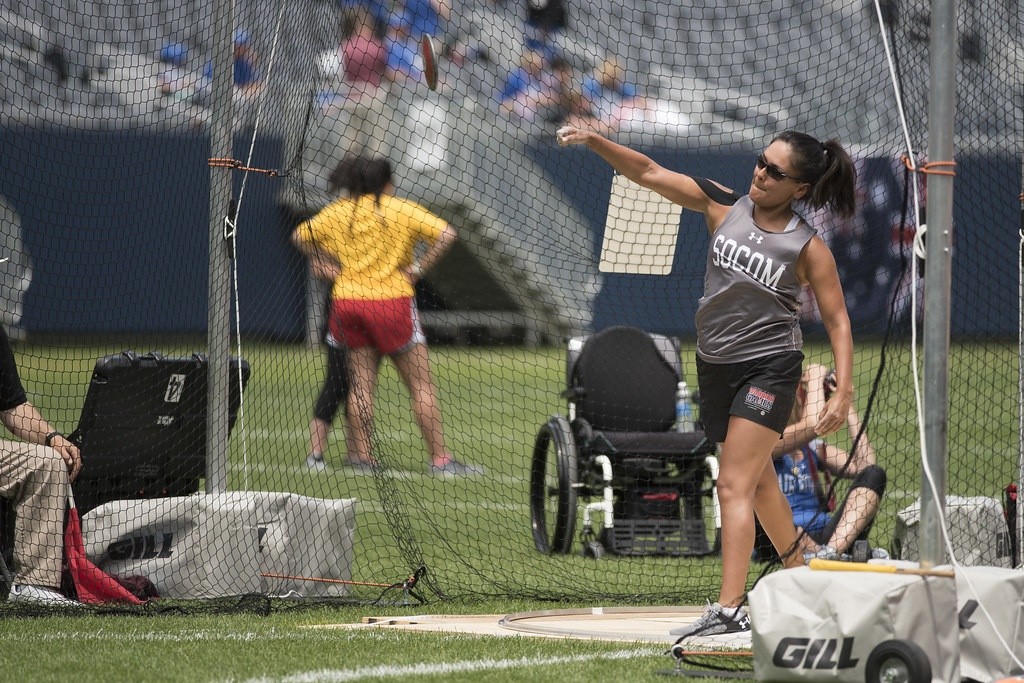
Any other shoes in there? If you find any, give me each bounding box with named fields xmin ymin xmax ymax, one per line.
xmin=432 ymin=461 xmax=486 ymax=479
xmin=6 ymin=585 xmax=82 ymax=609
xmin=346 ymin=456 xmax=385 ymax=475
xmin=804 ymin=544 xmax=851 ymax=565
xmin=307 ymin=454 xmax=326 ymax=471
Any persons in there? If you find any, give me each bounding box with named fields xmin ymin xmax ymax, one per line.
xmin=0 ymin=327 xmax=84 ymax=608
xmin=502 ymin=48 xmax=631 ymax=131
xmin=555 ymin=127 xmax=858 ymax=636
xmin=293 ymin=155 xmax=487 ymax=476
xmin=201 ymin=29 xmax=255 ymax=88
xmin=342 ymin=0 xmax=453 ymax=173
xmin=752 ymin=364 xmax=890 ymax=565
xmin=157 ymin=43 xmax=187 ymax=66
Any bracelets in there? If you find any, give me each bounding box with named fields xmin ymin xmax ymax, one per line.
xmin=45 ymin=430 xmax=63 ymax=447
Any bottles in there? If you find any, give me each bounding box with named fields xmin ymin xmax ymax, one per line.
xmin=676 ymin=382 xmax=694 ymax=435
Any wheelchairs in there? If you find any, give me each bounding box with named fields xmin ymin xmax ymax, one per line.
xmin=527 ymin=324 xmax=723 ymax=562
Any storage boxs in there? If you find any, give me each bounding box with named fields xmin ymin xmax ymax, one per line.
xmin=61 ymin=353 xmax=251 ymax=514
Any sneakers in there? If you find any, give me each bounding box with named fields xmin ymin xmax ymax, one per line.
xmin=669 ymin=598 xmax=751 ymax=636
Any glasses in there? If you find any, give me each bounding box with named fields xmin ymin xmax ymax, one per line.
xmin=756 ymin=153 xmax=803 ymax=182
